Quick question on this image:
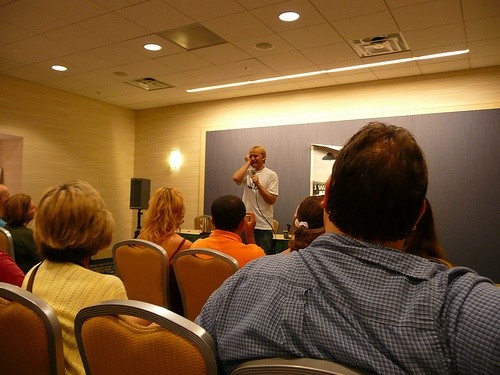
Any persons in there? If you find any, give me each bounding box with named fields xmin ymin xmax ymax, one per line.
xmin=0 ymin=186 xmax=443 ymax=375
xmin=194 ymin=122 xmax=500 ymax=375
xmin=234 ymin=146 xmax=279 ymax=255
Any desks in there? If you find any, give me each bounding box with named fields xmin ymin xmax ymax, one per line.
xmin=174 ymin=229 xmax=295 ymax=256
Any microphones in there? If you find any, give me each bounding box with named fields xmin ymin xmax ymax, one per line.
xmin=250 ymin=170 xmax=256 ymax=183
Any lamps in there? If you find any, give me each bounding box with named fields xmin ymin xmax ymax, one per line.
xmin=322 ymin=152 xmax=336 ymax=160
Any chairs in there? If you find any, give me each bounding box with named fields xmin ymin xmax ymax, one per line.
xmin=0 ymin=226 xmax=362 ymax=375
xmin=194 ymin=215 xmax=212 ymax=230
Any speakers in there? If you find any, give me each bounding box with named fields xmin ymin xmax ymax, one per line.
xmin=130 ymin=178 xmax=150 ymax=209
